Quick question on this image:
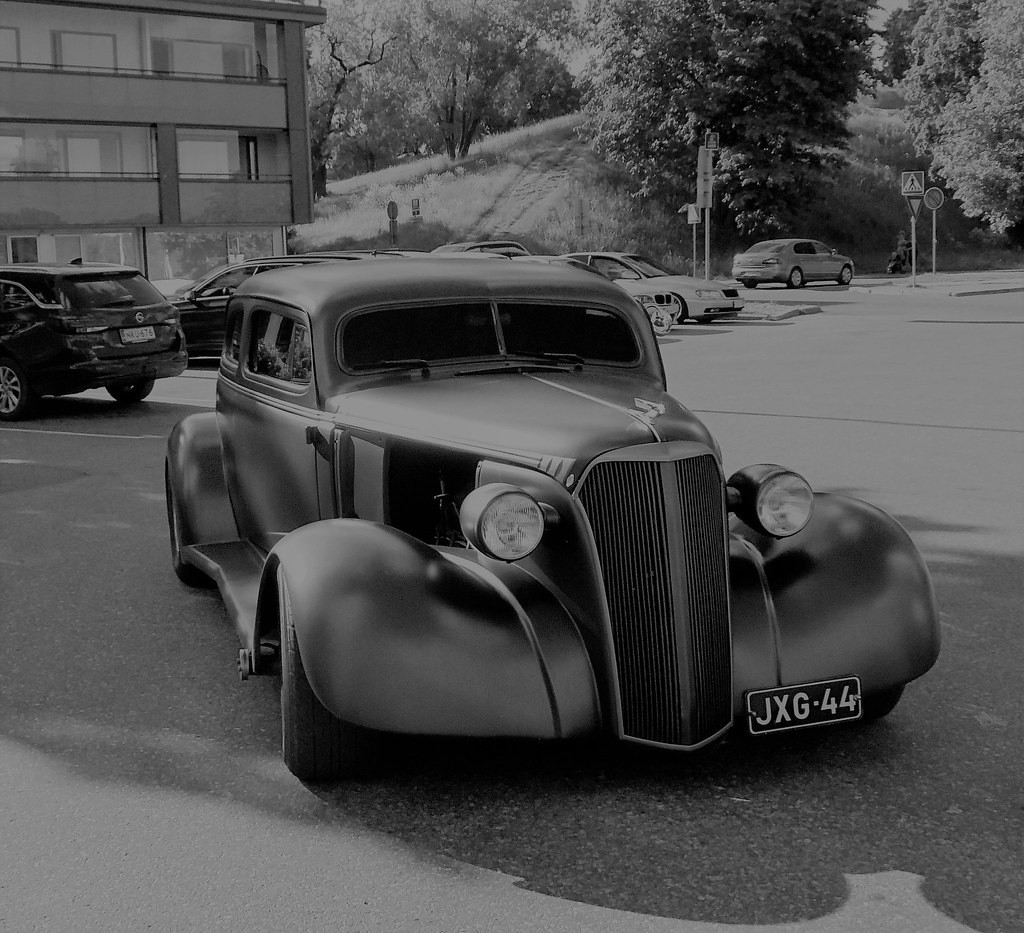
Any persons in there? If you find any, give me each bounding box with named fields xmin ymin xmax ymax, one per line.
xmin=888 ymin=230 xmax=919 ymax=274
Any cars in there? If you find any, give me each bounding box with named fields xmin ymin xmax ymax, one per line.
xmin=165 ymin=238 xmax=679 ymax=370
xmin=731 ymin=237 xmax=855 ymax=289
xmin=161 ymin=252 xmax=944 ymax=789
xmin=558 ymin=252 xmax=746 ymax=326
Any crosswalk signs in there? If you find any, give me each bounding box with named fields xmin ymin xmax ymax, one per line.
xmin=901 ymin=170 xmax=925 ymax=196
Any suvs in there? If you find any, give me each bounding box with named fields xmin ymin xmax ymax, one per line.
xmin=0 ymin=255 xmax=190 ymax=423
